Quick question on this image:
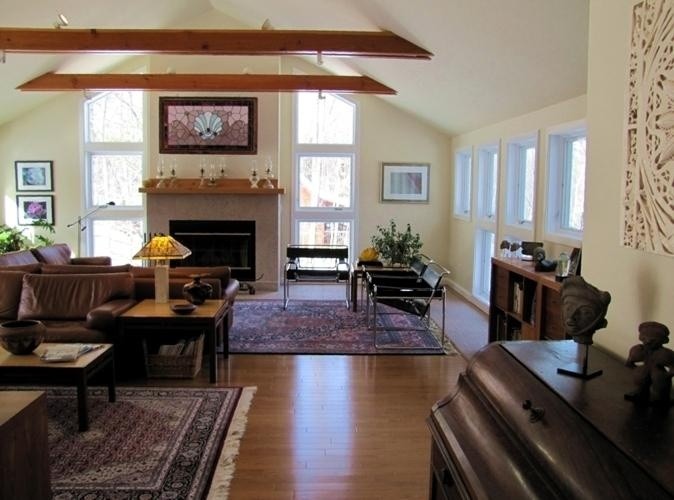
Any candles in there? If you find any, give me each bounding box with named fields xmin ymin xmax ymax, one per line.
xmin=250 ymin=158 xmax=257 ymax=172
xmin=266 ymin=156 xmax=272 ymax=170
xmin=170 ymin=156 xmax=176 ymax=171
xmin=158 ymin=159 xmax=164 ymax=172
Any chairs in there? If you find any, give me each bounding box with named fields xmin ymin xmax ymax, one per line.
xmin=355 ymin=253 xmax=433 ymax=330
xmin=368 ymin=262 xmax=449 ymax=349
xmin=282 ymin=243 xmax=350 ymax=311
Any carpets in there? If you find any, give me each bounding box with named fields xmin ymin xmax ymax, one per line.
xmin=213 ymin=298 xmax=459 ymax=357
xmin=1 ymin=382 xmax=256 ymax=497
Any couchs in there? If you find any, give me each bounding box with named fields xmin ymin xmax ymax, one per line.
xmin=0 ymin=271 xmax=134 ymax=344
xmin=0 ymin=243 xmax=239 ymax=344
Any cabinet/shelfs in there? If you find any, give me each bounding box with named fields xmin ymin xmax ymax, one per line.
xmin=487 ymin=256 xmax=570 ymax=346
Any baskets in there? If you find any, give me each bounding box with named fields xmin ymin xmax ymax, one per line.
xmin=141 ymin=326 xmax=205 ymax=379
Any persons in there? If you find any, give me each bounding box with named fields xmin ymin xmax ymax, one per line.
xmin=622 ymin=322 xmax=674 ymax=408
xmin=557 ymin=276 xmax=611 ymax=382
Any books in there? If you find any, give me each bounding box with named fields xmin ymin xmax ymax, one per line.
xmin=40 ymin=342 xmax=101 ymax=365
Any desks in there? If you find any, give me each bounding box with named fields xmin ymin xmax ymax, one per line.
xmin=0 ymin=389 xmax=48 ymax=500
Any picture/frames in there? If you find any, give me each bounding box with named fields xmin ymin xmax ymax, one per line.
xmin=380 ymin=161 xmax=431 ymax=205
xmin=157 ymin=96 xmax=259 ymax=155
xmin=14 ymin=160 xmax=53 ymax=193
xmin=15 ymin=194 xmax=54 ymax=227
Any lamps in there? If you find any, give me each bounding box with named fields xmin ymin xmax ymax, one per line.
xmin=132 ymin=233 xmax=192 ymax=304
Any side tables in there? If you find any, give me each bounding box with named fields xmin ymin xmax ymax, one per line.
xmin=118 ymin=298 xmax=233 ymax=384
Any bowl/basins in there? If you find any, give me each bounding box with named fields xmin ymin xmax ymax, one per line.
xmin=169 ymin=303 xmax=196 ymax=315
